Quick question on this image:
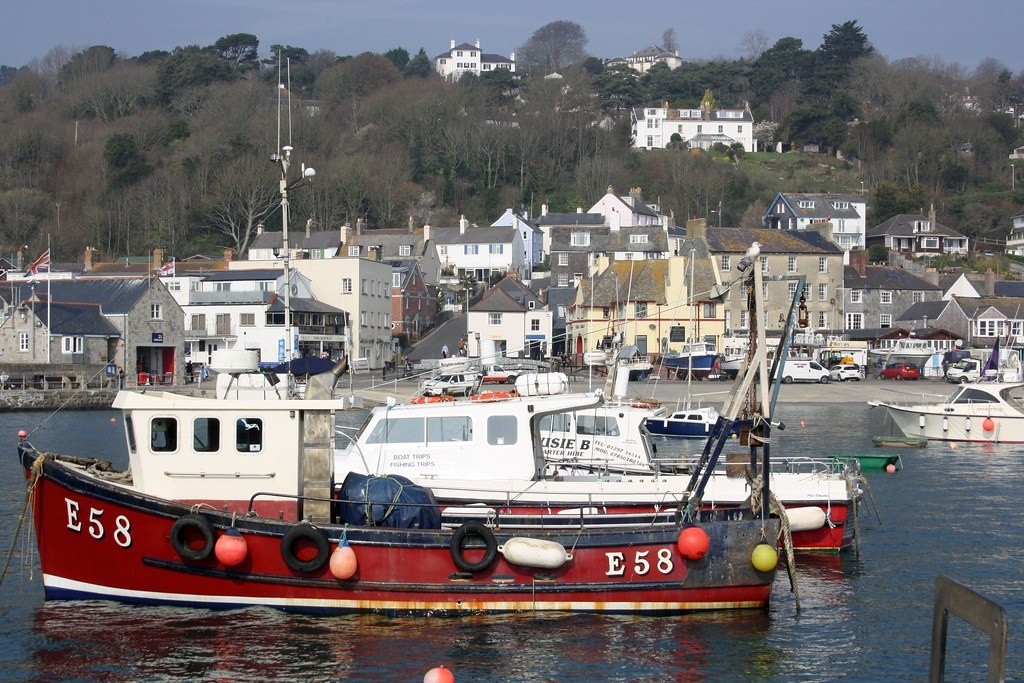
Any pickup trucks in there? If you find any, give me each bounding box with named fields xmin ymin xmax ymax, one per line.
xmin=476 ymin=363 xmax=518 ymax=384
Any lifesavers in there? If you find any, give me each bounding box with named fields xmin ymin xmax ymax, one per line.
xmin=470 ymin=392 xmax=521 ymax=400
xmin=280 ymin=524 xmax=330 ymax=572
xmin=411 ymin=396 xmax=455 ymax=404
xmin=169 ymin=515 xmax=216 ymax=561
xmin=450 ymin=523 xmax=498 ymax=572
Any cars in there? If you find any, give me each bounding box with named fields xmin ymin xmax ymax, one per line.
xmin=880 ymin=362 xmax=918 ymax=380
xmin=829 ymin=365 xmax=860 ymax=383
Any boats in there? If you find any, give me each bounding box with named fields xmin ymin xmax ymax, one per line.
xmin=331 ymin=258 xmax=864 ymax=555
xmin=719 ymin=333 xmax=748 ymax=378
xmin=871 ymin=435 xmax=928 ymax=447
xmin=869 ymin=320 xmax=939 ymax=369
xmin=14 ymin=49 xmax=807 ymax=618
xmin=866 ymin=303 xmax=1024 ymax=444
xmin=826 ymin=454 xmax=902 ymax=471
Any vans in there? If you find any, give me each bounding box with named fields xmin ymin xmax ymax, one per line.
xmin=770 ymin=359 xmax=829 ymax=384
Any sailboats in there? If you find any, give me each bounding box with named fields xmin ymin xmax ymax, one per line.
xmin=609 ymin=259 xmax=654 ymax=381
xmin=641 ymin=244 xmax=736 ymax=440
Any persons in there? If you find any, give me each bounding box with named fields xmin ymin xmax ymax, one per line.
xmin=458 ymin=338 xmax=465 ymax=354
xmin=390 ymin=351 xmax=396 ymax=372
xmin=441 ymin=344 xmax=449 ymax=358
xmin=185 ymin=360 xmax=193 ymax=383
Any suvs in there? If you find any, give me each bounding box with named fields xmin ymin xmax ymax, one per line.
xmin=422 ymin=372 xmax=480 ymax=397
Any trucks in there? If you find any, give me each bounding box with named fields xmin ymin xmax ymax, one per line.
xmin=946 ymin=358 xmax=1019 ymax=383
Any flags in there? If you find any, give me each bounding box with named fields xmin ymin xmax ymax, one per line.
xmin=23 ymin=251 xmax=49 ymax=277
xmin=157 ymin=261 xmax=174 ymax=277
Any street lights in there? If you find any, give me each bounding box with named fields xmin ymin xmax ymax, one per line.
xmin=462 ymin=287 xmax=472 ymax=311
